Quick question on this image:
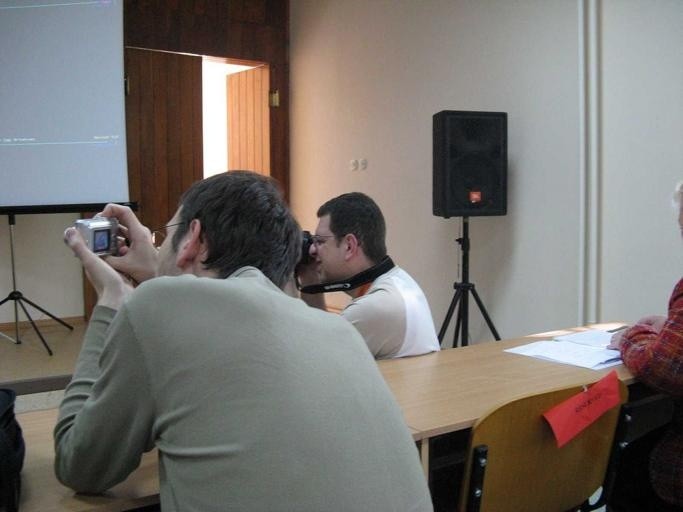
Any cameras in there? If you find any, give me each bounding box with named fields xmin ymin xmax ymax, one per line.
xmin=62 ymin=217 xmax=120 ymax=258
xmin=295 ymin=230 xmax=313 ymax=267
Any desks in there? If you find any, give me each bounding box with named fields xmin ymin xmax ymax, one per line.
xmin=15 ymin=404 xmax=421 ymax=511
xmin=376 ymin=322 xmax=682 ymax=509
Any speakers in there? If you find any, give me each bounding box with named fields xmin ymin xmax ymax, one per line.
xmin=433 ymin=110 xmax=508 ymax=216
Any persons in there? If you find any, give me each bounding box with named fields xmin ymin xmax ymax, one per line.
xmin=97 ymin=235 xmax=106 ymax=249
xmin=299 ymin=192 xmax=441 ymax=360
xmin=53 ymin=169 xmax=435 ymax=512
xmin=608 ymin=183 xmax=683 ymax=512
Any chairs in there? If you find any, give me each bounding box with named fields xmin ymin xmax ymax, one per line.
xmin=462 ymin=379 xmax=630 ymax=509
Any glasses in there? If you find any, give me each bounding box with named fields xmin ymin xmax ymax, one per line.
xmin=308 ymin=233 xmax=335 ymax=246
xmin=151 ymin=221 xmax=183 ymax=250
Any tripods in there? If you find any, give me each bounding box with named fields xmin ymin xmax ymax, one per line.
xmin=0 ymin=215 xmax=74 ymax=357
xmin=438 ymin=216 xmax=500 ymax=347
